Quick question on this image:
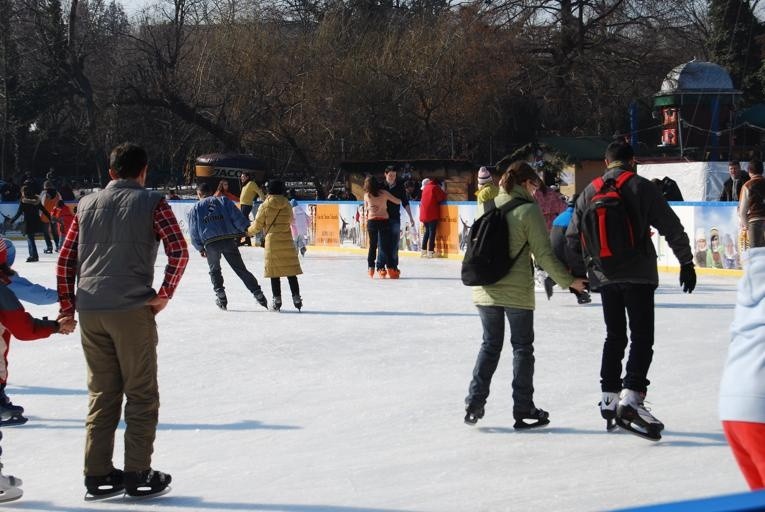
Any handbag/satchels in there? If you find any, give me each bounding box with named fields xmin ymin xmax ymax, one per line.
xmin=259 ymin=236 xmax=265 ymax=247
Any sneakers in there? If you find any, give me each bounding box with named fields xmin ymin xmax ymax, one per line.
xmin=512 ymin=406 xmax=549 ymax=421
xmin=258 ymin=296 xmax=267 ymax=306
xmin=466 ymin=402 xmax=485 ymax=418
xmin=273 ymin=297 xmax=283 ymax=307
xmin=1 ymin=406 xmax=28 ymax=425
xmin=601 ymin=389 xmax=665 ymax=433
xmin=292 ymin=296 xmax=302 ymax=305
xmin=127 ymin=469 xmax=172 ymax=495
xmin=84 ymin=468 xmax=125 ymax=493
xmin=368 ymin=266 xmax=400 ymax=277
xmin=215 ymin=297 xmax=226 ymax=306
xmin=422 ymin=249 xmax=433 ymax=257
xmin=1 ymin=475 xmax=22 ymax=502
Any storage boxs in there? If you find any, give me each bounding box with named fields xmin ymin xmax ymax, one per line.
xmin=446 ymin=194 xmax=468 ymax=201
xmin=445 ymin=182 xmax=469 ymax=194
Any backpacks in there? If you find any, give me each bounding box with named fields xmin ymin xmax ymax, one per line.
xmin=460 ymin=197 xmax=534 ymax=285
xmin=579 ymin=169 xmax=633 ymax=277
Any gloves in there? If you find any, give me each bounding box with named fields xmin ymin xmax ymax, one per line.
xmin=680 ymin=265 xmax=696 ymax=293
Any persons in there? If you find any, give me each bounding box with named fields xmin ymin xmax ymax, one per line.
xmin=651 ymin=177 xmax=683 ymax=201
xmin=718 ymin=246 xmax=765 ymax=492
xmin=0 ymin=169 xmax=87 ymax=262
xmin=739 ymin=160 xmax=765 ymax=247
xmin=167 ymin=173 xmax=265 ymax=248
xmin=0 ymin=238 xmax=58 ymax=416
xmin=474 ymin=168 xmax=590 ymax=305
xmin=328 ymin=167 xmax=448 ymax=280
xmin=57 ymin=143 xmax=189 ymax=489
xmin=720 ymin=160 xmax=751 ymax=201
xmin=190 ymin=180 xmax=311 ymax=313
xmin=695 ymin=228 xmax=735 ymax=270
xmin=465 ymin=161 xmax=589 ymax=421
xmin=0 ymin=238 xmax=78 ymax=492
xmin=565 ymin=141 xmax=697 ymax=432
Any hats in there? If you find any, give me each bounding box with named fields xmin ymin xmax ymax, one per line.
xmin=0 ymin=239 xmax=16 ymax=268
xmin=419 ymin=178 xmax=429 ymax=191
xmin=478 ymin=164 xmax=492 ymax=187
